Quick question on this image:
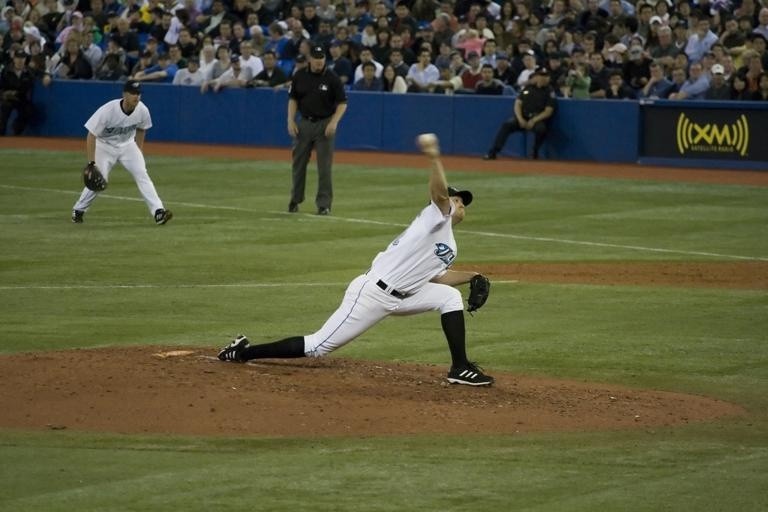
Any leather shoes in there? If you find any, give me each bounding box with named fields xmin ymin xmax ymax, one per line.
xmin=289 ymin=204 xmax=298 ymax=212
xmin=318 ymin=206 xmax=330 ymax=215
xmin=483 ymin=154 xmax=495 ymax=160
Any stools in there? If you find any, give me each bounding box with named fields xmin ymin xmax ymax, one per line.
xmin=515 ymin=128 xmax=542 ymax=160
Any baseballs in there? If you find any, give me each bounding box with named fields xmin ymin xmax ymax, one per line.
xmin=419 ymin=133 xmax=437 ymax=149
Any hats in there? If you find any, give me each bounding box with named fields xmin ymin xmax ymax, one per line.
xmin=122 ymin=79 xmax=144 ymax=94
xmin=2 ymin=1 xmax=725 ymax=77
xmin=432 ymin=185 xmax=473 ymax=206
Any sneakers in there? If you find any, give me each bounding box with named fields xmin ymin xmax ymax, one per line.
xmin=218 ymin=335 xmax=250 ymax=363
xmin=71 ymin=209 xmax=85 ymax=223
xmin=155 ymin=209 xmax=173 ymax=224
xmin=446 ymin=362 xmax=496 ymax=386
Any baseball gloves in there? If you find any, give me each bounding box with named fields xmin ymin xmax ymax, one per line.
xmin=466 ymin=275 xmax=490 ymax=312
xmin=83 ymin=161 xmax=108 ymax=192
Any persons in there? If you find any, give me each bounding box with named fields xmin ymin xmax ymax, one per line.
xmin=1 ymin=1 xmax=768 ymax=101
xmin=216 ymin=129 xmax=497 ymax=387
xmin=480 ymin=64 xmax=560 ymax=162
xmin=285 ymin=44 xmax=348 ymax=217
xmin=1 ymin=49 xmax=40 ymax=136
xmin=70 ymin=79 xmax=175 ymax=226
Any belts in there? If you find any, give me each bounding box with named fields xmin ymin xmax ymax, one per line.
xmin=300 ymin=114 xmax=332 ymax=124
xmin=366 ymin=269 xmax=407 ymax=299
